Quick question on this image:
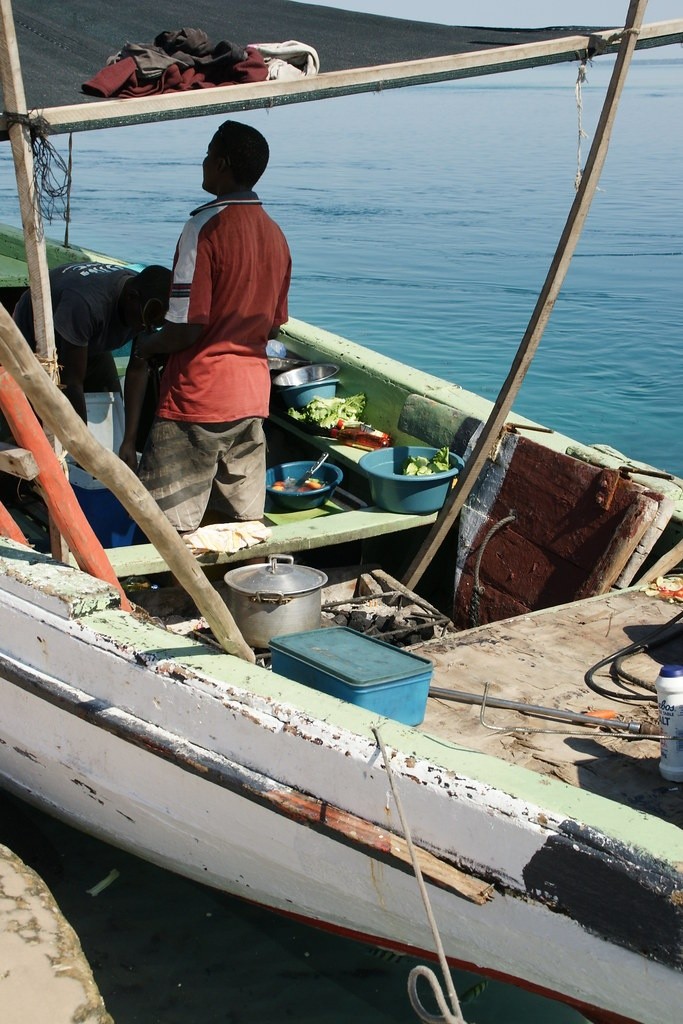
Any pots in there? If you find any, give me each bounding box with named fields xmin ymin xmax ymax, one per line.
xmin=224 ymin=555 xmax=329 ymax=649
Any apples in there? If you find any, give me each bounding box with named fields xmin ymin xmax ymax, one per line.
xmin=271 ymin=480 xmax=321 ymax=492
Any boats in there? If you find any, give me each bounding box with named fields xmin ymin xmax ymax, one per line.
xmin=0 ymin=0 xmax=683 ymax=1023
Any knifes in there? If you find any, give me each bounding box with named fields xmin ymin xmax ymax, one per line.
xmin=296 ymin=452 xmax=330 ymax=487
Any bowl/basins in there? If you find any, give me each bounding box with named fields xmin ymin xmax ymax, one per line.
xmin=265 ymin=461 xmax=344 ymax=509
xmin=278 ymin=379 xmax=341 ymax=411
xmin=268 ymin=357 xmax=312 ymax=379
xmin=271 ymin=363 xmax=340 ymax=389
xmin=358 ymin=444 xmax=466 ymax=514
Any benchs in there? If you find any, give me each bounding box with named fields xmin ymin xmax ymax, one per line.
xmin=261 ymin=400 xmax=384 ymax=470
xmin=40 ymin=503 xmax=442 ymax=577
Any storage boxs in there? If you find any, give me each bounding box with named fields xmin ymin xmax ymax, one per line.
xmin=267 ymin=627 xmax=433 ymax=726
xmin=63 ymin=391 xmax=143 ymax=490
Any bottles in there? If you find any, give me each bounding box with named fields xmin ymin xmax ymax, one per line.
xmin=330 ymin=429 xmax=383 ymax=452
xmin=657 ymin=663 xmax=683 ymax=783
xmin=336 ymin=419 xmax=394 ymax=445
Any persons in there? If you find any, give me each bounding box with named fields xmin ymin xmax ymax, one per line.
xmin=129 ymin=122 xmax=291 ymax=586
xmin=12 ymin=261 xmax=173 ymax=474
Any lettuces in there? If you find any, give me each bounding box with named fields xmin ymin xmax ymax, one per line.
xmin=402 ymin=446 xmax=452 ymax=475
xmin=287 ymin=392 xmax=366 ymax=429
